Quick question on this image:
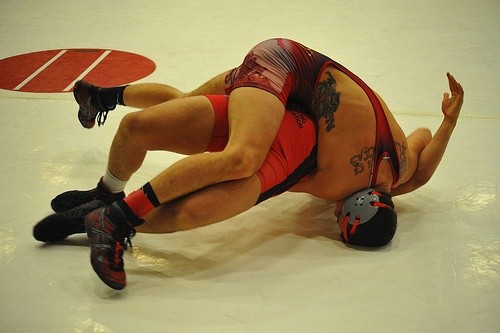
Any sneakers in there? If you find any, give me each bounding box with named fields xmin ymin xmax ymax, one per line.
xmin=50 ymin=176 xmax=126 ymax=213
xmin=84 ymin=200 xmax=147 ymax=292
xmin=32 ymin=200 xmax=108 ymax=244
xmin=73 ymin=79 xmax=100 ymax=129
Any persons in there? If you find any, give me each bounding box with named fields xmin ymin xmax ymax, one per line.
xmin=31 ymin=72 xmax=464 ymax=243
xmin=73 ymin=39 xmax=433 ymax=290
